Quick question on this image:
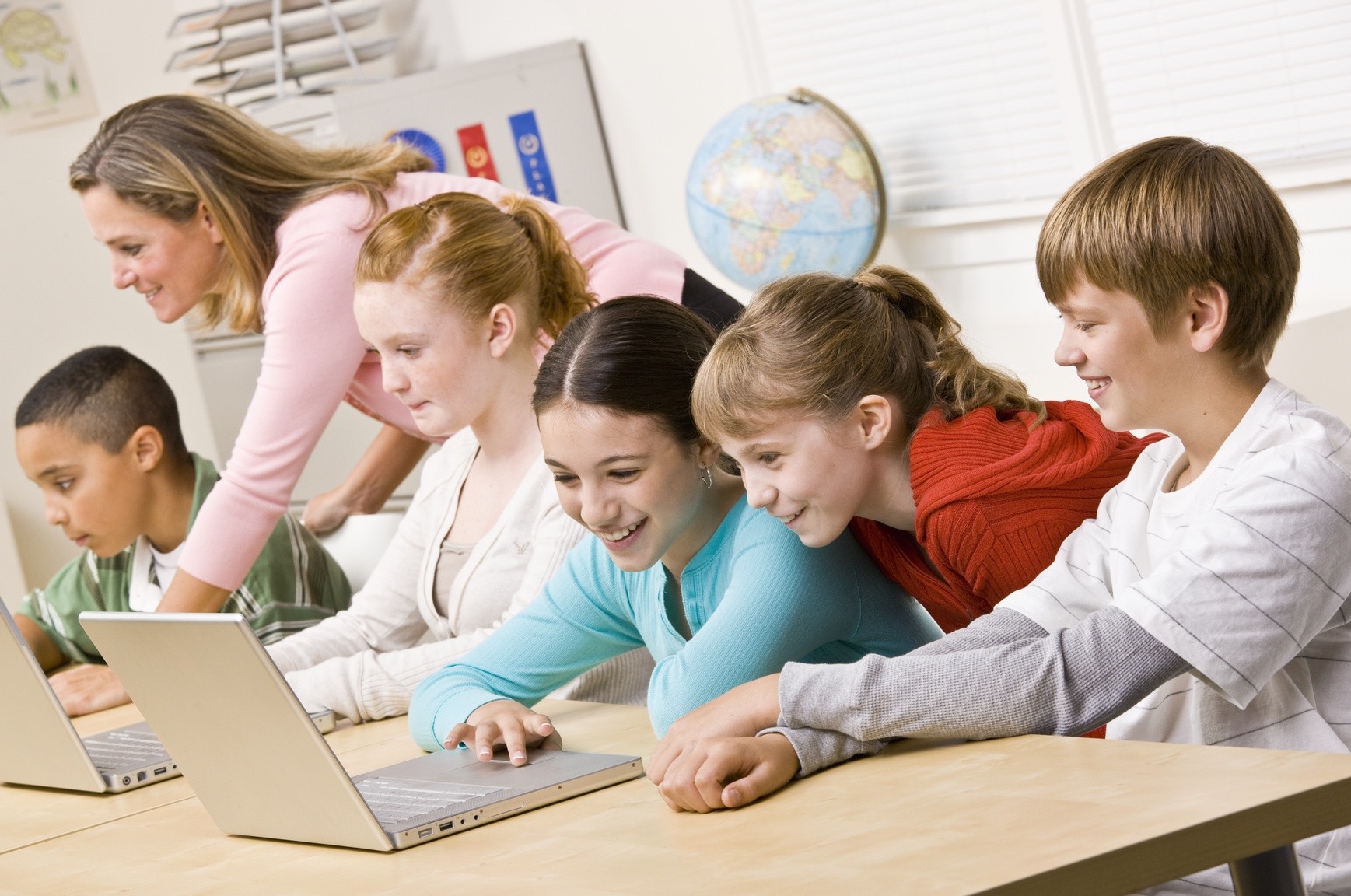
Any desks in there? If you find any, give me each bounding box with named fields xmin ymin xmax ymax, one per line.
xmin=1 ymin=696 xmax=1350 ymax=896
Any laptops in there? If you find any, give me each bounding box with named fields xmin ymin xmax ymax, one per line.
xmin=77 ymin=612 xmax=644 ymax=854
xmin=0 ymin=598 xmax=337 ymax=794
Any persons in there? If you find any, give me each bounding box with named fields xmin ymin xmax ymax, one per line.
xmin=11 ymin=93 xmax=1351 ymax=896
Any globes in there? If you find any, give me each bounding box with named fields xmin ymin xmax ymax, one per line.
xmin=686 ymin=86 xmax=887 ymax=295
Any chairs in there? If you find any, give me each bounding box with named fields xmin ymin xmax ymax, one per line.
xmin=314 ymin=512 xmax=403 ymax=596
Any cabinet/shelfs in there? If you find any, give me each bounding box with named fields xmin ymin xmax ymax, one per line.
xmin=168 ymin=0 xmax=625 ymax=510
